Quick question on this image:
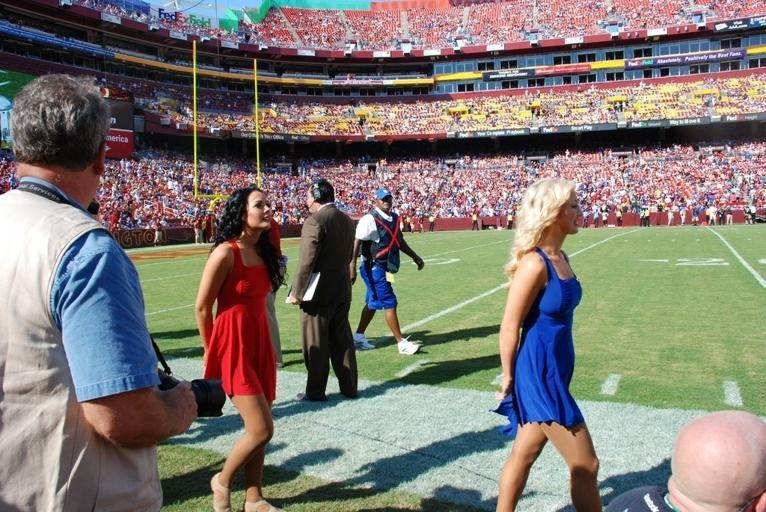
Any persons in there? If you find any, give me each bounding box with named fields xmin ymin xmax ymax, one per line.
xmin=195 ymin=188 xmax=284 ymax=512
xmin=488 ymin=180 xmax=603 ymax=512
xmin=349 ymin=188 xmax=425 ymax=356
xmin=2 ymin=1 xmax=764 ymax=248
xmin=289 ymin=180 xmax=357 ymax=401
xmin=0 ymin=73 xmax=199 ymax=512
xmin=266 ymin=220 xmax=285 ymax=368
xmin=604 ymin=410 xmax=766 ymax=512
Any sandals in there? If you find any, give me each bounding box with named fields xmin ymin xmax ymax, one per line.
xmin=211 ymin=472 xmax=285 ymax=512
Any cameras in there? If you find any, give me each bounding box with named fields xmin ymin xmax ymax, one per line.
xmin=157 ymin=369 xmax=226 ymax=417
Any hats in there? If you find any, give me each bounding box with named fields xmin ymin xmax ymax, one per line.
xmin=374 ymin=187 xmax=392 ymax=199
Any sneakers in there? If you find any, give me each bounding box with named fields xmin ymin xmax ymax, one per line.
xmin=352 ymin=337 xmax=376 ymax=351
xmin=398 ymin=339 xmax=422 ymax=354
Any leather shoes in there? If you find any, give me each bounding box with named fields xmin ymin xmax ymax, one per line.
xmin=297 ymin=393 xmax=307 ymax=400
